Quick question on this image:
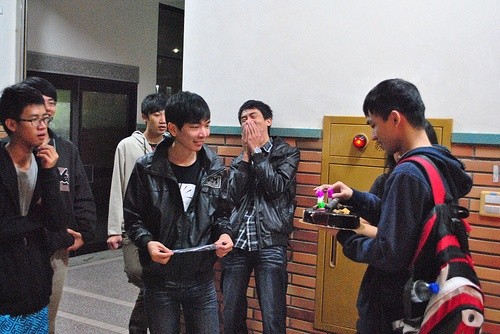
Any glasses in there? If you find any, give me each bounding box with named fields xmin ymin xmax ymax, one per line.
xmin=16 ymin=114 xmax=53 ymax=127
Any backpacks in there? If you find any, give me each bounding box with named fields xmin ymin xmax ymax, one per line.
xmin=382 ymin=154 xmax=485 ymax=334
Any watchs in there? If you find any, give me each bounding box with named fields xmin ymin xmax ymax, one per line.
xmin=251 ymin=148 xmax=262 ymax=156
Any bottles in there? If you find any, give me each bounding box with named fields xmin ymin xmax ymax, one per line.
xmin=411 ymin=281 xmax=439 ymax=304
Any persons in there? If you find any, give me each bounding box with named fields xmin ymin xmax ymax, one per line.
xmin=124 ymin=91 xmax=235 ymax=334
xmin=328 ymin=79 xmax=473 ymax=334
xmin=107 ymin=94 xmax=172 ymax=334
xmin=0 ymin=77 xmax=96 ymax=334
xmin=0 ymin=85 xmax=68 ymax=334
xmin=337 ymin=120 xmax=438 ymax=334
xmin=220 ymin=100 xmax=300 ymax=334
